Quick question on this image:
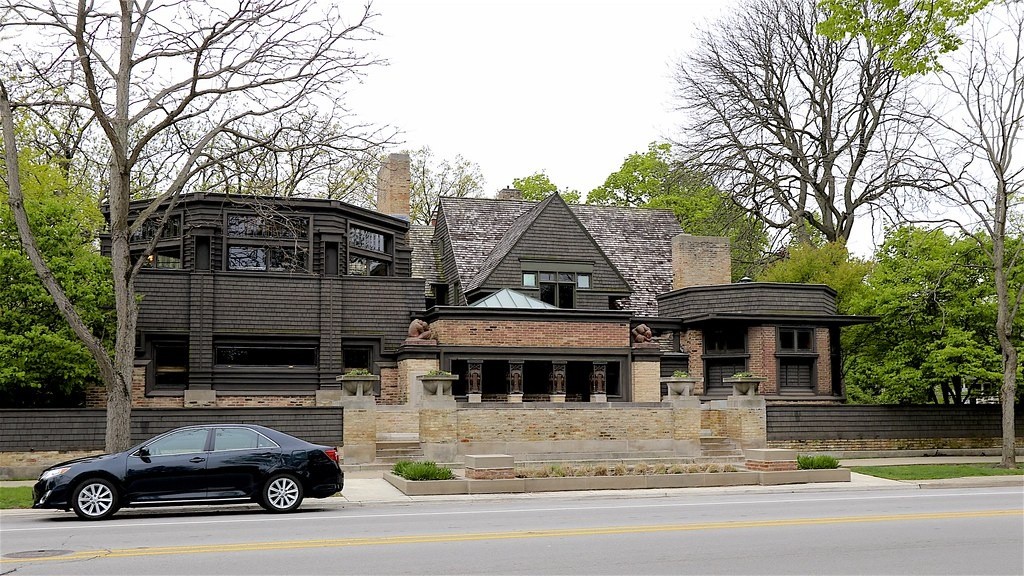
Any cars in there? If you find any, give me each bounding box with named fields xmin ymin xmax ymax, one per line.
xmin=30 ymin=423 xmax=343 ymax=521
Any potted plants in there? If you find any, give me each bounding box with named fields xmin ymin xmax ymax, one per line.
xmin=335 ymin=368 xmax=381 ymax=394
xmin=416 ymin=370 xmax=460 ymax=394
xmin=660 ymin=370 xmax=704 ymax=396
xmin=723 ymin=373 xmax=768 ymax=395
